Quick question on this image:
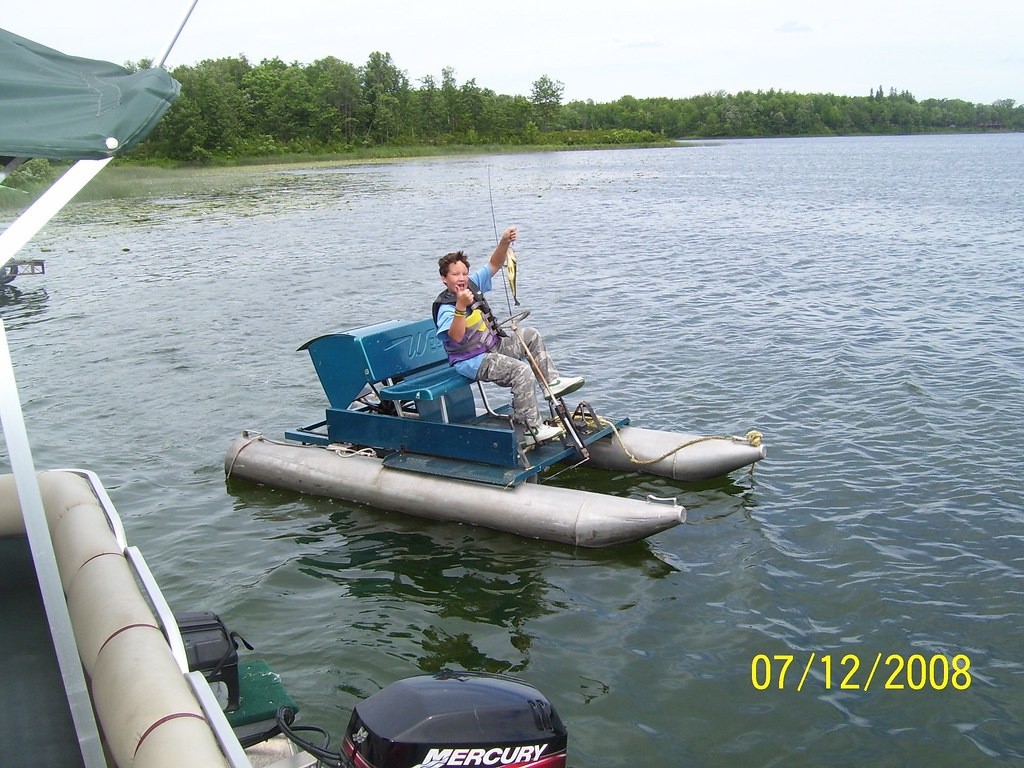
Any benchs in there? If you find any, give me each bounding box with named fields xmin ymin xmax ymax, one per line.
xmin=355 ymin=318 xmax=511 ymax=428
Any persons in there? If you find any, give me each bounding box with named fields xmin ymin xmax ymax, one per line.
xmin=431 ymin=227 xmax=584 ymax=445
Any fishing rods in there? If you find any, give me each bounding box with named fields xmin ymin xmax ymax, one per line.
xmin=487 ymin=165 xmax=513 ymax=324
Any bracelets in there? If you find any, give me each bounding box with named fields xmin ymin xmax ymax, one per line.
xmin=454 ymin=308 xmax=466 ymax=316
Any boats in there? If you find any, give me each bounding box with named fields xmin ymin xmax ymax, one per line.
xmin=225 ymin=313 xmax=766 ymax=545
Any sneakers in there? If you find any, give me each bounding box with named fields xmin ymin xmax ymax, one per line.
xmin=543 ymin=376 xmax=585 ymax=400
xmin=524 ymin=424 xmax=562 ymax=445
